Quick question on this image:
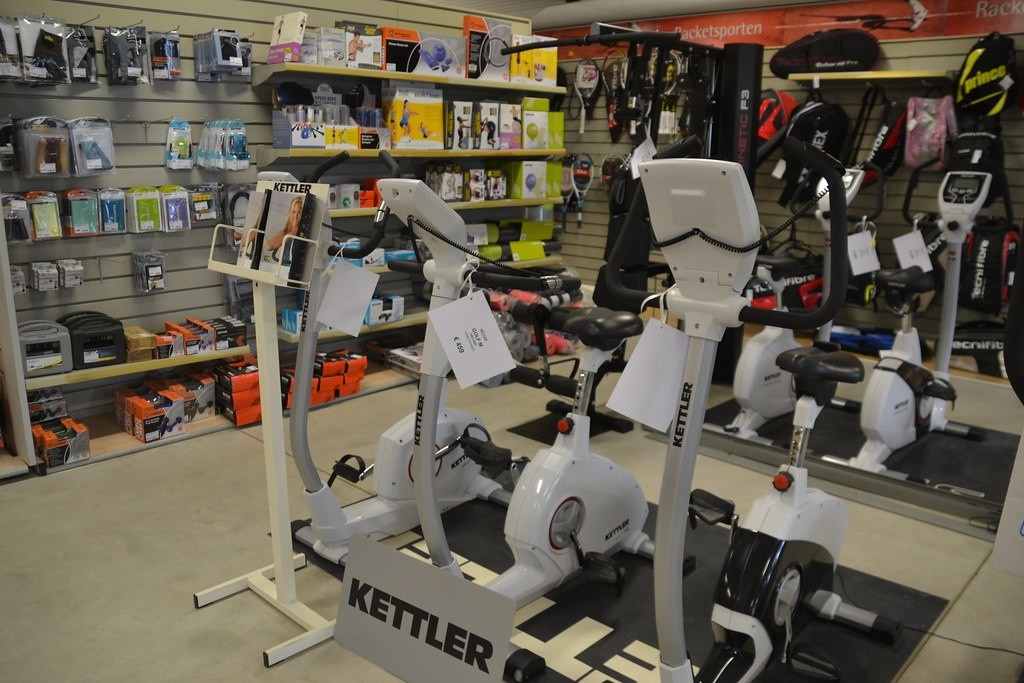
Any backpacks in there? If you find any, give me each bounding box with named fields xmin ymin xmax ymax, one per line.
xmin=895 ymin=211 xmax=950 ymax=312
xmin=782 ymin=89 xmax=849 ymax=190
xmin=848 ymin=84 xmax=906 ymax=190
xmin=955 ymin=31 xmax=1018 ymax=117
xmin=905 ymin=84 xmax=958 ymax=172
xmin=950 ymin=118 xmax=1004 ymax=208
xmin=957 ymin=217 xmax=1020 ymax=313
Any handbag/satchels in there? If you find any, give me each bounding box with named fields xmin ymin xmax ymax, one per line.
xmin=769 ymin=28 xmax=880 ymax=79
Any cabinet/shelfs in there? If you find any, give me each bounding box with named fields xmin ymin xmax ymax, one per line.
xmin=251 ymin=62 xmax=567 ymax=416
xmin=25 ymin=341 xmax=252 ymax=476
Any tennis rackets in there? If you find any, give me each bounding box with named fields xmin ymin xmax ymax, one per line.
xmin=556 ymin=151 xmax=630 ymax=234
xmin=575 ymin=46 xmax=686 ymax=137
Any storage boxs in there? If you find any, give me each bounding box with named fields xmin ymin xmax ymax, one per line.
xmin=463 ymin=218 xmax=555 ymax=262
xmin=27 ymin=386 xmax=90 ymax=469
xmin=267 ymin=11 xmax=565 ymax=208
xmin=361 ymin=333 xmax=424 ymax=380
xmin=121 ymin=315 xmax=246 ymax=362
xmin=331 ymin=230 xmax=416 ymax=268
xmin=113 ymin=348 xmax=368 ymax=444
xmin=280 ymin=289 xmax=405 ymax=335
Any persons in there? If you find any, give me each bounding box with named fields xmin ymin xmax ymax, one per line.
xmin=347 ymin=31 xmax=371 ymax=61
xmin=299 ymin=14 xmax=308 ymax=35
xmin=329 ymin=126 xmax=340 ymax=144
xmin=515 ymin=42 xmax=523 ymax=75
xmin=428 ymin=172 xmax=501 ymax=198
xmin=268 ymin=197 xmax=304 ymax=268
xmin=456 ymin=115 xmax=471 ymax=149
xmin=275 ymin=13 xmax=288 ymax=44
xmin=388 ymin=99 xmax=433 ymax=143
xmin=481 ymin=116 xmax=496 ymax=148
